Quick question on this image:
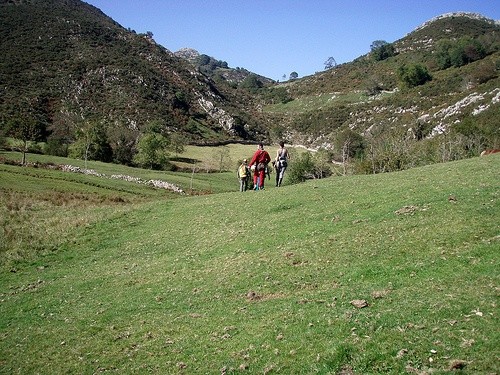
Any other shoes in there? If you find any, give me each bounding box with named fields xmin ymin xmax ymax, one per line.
xmin=253 ymin=184 xmax=257 ymax=190
xmin=276 ymin=181 xmax=278 ymax=186
xmin=260 ymin=186 xmax=264 ymax=189
xmin=278 ymin=183 xmax=281 ymax=187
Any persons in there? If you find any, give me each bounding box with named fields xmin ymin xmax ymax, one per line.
xmin=247 ymin=143 xmax=271 ymax=191
xmin=275 ymin=141 xmax=290 ymax=187
xmin=237 ymin=159 xmax=252 ymax=191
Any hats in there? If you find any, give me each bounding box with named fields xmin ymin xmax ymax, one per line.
xmin=243 ymin=159 xmax=247 ymax=163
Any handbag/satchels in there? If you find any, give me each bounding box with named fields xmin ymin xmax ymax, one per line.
xmin=257 ymin=162 xmax=267 ymax=170
xmin=272 ymin=158 xmax=287 ymax=172
